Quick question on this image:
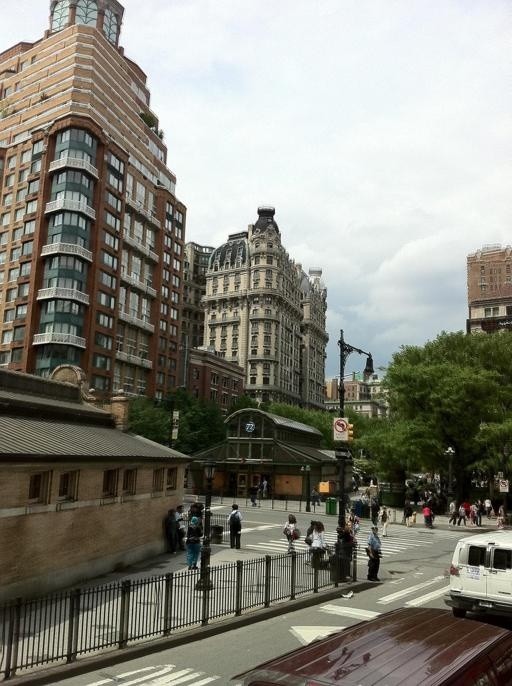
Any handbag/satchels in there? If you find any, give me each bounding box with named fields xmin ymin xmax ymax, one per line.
xmin=284 ymin=528 xmax=291 ymax=535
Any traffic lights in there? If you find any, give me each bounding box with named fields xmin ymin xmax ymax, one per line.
xmin=348 ymin=423 xmax=353 ymax=443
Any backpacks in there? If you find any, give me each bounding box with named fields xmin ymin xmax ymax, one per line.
xmin=230 ymin=511 xmax=241 ymax=531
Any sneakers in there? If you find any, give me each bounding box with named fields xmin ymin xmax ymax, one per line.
xmin=188 ymin=563 xmax=198 ymax=570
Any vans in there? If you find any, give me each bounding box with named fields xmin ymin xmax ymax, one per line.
xmin=228 ymin=607 xmax=512 ymax=686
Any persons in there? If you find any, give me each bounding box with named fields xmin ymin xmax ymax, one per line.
xmin=247 ymin=483 xmax=259 ymax=507
xmin=304 ymin=477 xmax=391 ymax=582
xmin=165 ymin=509 xmax=180 ymax=554
xmin=283 ymin=513 xmax=298 ymax=551
xmin=175 ymin=505 xmax=187 ymax=539
xmin=185 ymin=502 xmax=205 ymax=570
xmin=447 ymin=495 xmax=506 ymax=530
xmin=403 ymin=502 xmax=413 ymax=527
xmin=226 ymin=504 xmax=243 ymax=549
xmin=422 ymin=502 xmax=432 ymax=527
xmin=261 ymin=477 xmax=269 ymax=498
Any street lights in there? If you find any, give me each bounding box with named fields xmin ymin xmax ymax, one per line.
xmin=195 ymin=462 xmax=217 ymax=590
xmin=443 ymin=446 xmax=457 ymax=517
xmin=336 ymin=329 xmax=374 ymax=576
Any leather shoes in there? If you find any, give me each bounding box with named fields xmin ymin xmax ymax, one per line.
xmin=367 ymin=576 xmax=379 ymax=581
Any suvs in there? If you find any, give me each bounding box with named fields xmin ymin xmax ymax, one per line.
xmin=443 ymin=529 xmax=512 ymax=620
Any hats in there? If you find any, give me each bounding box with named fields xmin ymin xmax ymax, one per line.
xmin=370 ymin=525 xmax=379 ymax=530
xmin=191 ymin=517 xmax=199 ymax=525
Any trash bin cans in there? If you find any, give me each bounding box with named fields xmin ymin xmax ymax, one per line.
xmin=211 ymin=525 xmax=223 ymax=544
xmin=326 ymin=497 xmax=336 ymax=515
